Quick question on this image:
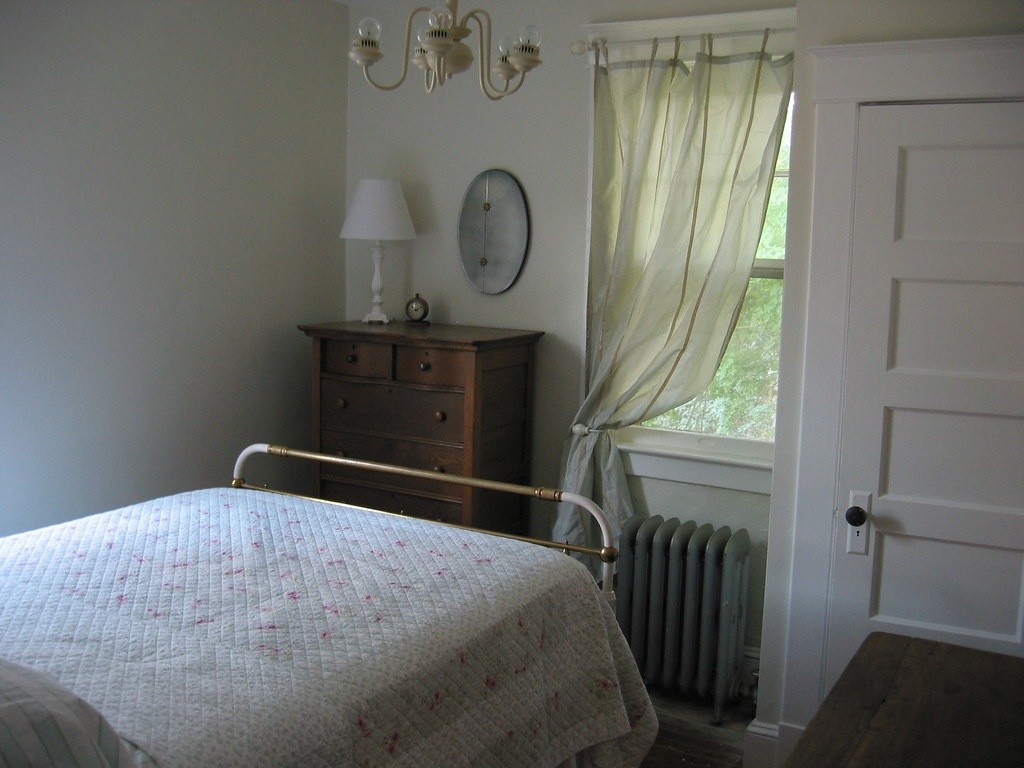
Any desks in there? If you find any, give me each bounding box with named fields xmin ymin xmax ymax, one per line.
xmin=785 ymin=631 xmax=1024 ymax=768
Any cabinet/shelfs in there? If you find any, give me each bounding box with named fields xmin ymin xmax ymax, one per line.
xmin=298 ymin=317 xmax=548 ymax=536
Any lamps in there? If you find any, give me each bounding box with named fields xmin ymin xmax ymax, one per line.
xmin=348 ymin=0 xmax=543 ymax=102
xmin=339 ymin=178 xmax=418 ymax=317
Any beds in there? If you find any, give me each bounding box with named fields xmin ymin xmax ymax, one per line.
xmin=0 ymin=442 xmax=661 ymax=768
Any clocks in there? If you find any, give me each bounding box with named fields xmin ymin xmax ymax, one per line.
xmin=405 ymin=293 xmax=431 ymax=325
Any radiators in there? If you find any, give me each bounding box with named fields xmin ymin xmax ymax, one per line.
xmin=615 ymin=511 xmax=759 ymax=725
